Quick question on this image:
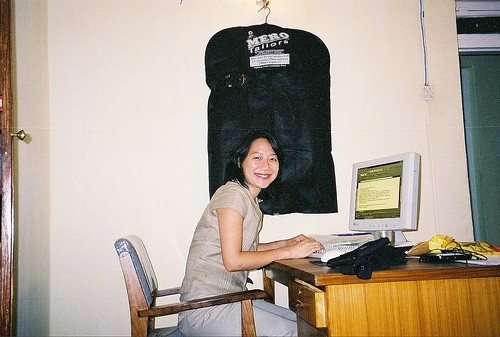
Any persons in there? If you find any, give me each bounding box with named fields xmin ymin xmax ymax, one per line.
xmin=177 ymin=132 xmax=326 ymax=337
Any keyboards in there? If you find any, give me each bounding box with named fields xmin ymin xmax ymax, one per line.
xmin=308 ymin=244 xmax=361 ymax=260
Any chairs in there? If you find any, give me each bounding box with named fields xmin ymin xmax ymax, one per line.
xmin=113 ymin=235 xmax=271 ymax=337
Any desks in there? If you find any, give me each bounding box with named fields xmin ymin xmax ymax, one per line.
xmin=260 ymin=248 xmax=500 ymax=337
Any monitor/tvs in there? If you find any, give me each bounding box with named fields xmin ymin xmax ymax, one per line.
xmin=349 ymin=152 xmax=420 ymax=232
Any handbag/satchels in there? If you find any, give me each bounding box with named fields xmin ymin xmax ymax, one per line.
xmin=327 ymin=237 xmax=407 ymax=280
